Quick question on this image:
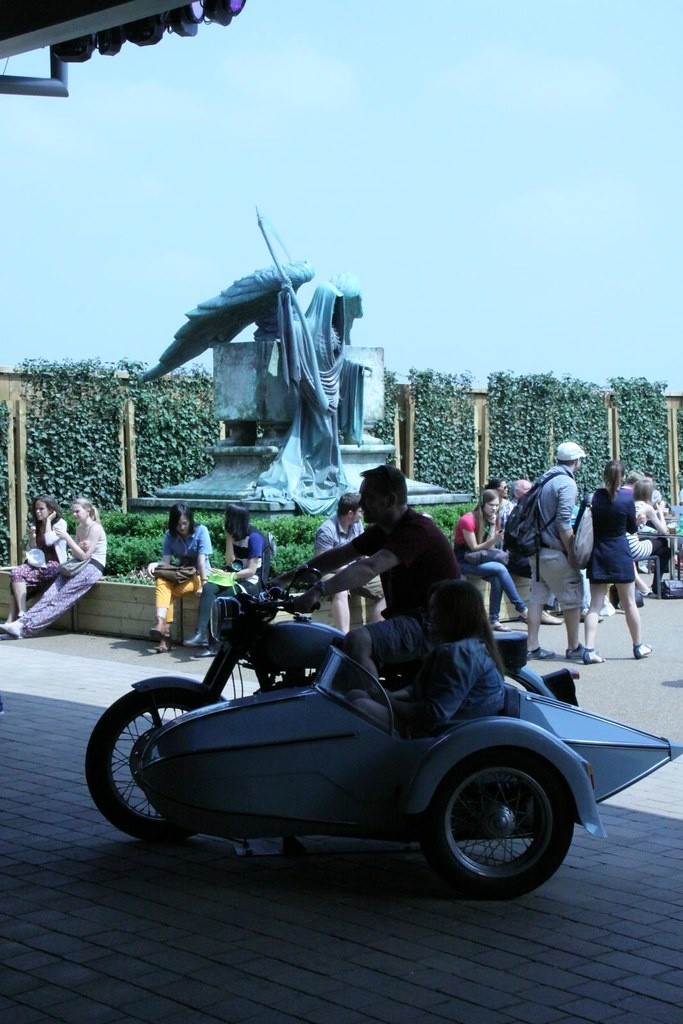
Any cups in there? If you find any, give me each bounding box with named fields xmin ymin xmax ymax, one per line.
xmin=663 ymin=508 xmax=670 ymax=517
xmin=667 ymin=525 xmax=676 ymax=534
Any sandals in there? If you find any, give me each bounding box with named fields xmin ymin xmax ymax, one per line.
xmin=634 ymin=645 xmax=651 ymax=659
xmin=582 ymin=649 xmax=605 ymax=664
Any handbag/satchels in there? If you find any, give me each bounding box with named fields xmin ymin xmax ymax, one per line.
xmin=465 ymin=548 xmax=509 ymax=566
xmin=568 ymin=493 xmax=594 ymax=569
xmin=207 ymin=570 xmax=238 ymax=586
xmin=59 ymin=557 xmax=90 ymax=578
xmin=153 ymin=564 xmax=196 ymax=583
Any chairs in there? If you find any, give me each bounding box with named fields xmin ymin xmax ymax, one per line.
xmin=633 ymin=555 xmax=661 ymax=600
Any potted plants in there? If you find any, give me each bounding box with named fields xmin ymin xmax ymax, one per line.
xmin=0 ymin=564 xmax=372 ymax=656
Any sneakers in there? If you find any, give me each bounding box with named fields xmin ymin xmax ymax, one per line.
xmin=527 ymin=647 xmax=555 ymax=661
xmin=566 ymin=643 xmax=586 ymax=659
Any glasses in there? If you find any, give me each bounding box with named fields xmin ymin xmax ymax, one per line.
xmin=503 ymin=485 xmax=509 ymax=490
xmin=357 ymin=509 xmax=364 ymax=514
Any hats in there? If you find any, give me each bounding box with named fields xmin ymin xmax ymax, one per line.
xmin=557 ymin=440 xmax=586 ymax=461
xmin=26 ymin=548 xmax=47 ymax=569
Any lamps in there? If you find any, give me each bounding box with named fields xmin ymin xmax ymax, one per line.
xmin=52 ymin=0 xmax=247 ymax=62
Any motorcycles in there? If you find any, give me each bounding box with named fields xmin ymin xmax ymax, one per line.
xmin=84 ymin=533 xmax=683 ymax=905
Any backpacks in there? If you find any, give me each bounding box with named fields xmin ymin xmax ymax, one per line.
xmin=503 ymin=472 xmax=564 ymax=558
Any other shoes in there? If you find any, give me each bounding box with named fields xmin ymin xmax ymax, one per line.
xmin=0 ymin=624 xmax=21 ymax=636
xmin=541 ymin=611 xmax=565 ymax=623
xmin=0 ymin=632 xmax=23 ymax=639
xmin=653 ymin=581 xmax=671 ymax=599
xmin=154 ymin=642 xmax=171 ymax=652
xmin=635 ymin=585 xmax=652 ymax=597
xmin=150 ymin=623 xmax=170 ymax=638
xmin=580 ymin=608 xmax=604 ymax=623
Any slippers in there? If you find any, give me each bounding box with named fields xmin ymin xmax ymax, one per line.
xmin=492 ymin=622 xmax=510 ymax=632
xmin=519 ymin=614 xmax=528 ymax=625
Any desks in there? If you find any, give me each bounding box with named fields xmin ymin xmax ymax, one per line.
xmin=638 ymin=533 xmax=683 ymax=580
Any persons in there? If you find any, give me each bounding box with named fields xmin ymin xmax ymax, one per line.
xmin=584 ymin=460 xmax=653 ymax=663
xmin=344 ymin=582 xmax=506 ymax=736
xmin=146 ymin=503 xmax=213 ymax=652
xmin=621 ymin=469 xmax=683 ymax=597
xmin=313 ymin=492 xmax=385 ymax=633
xmin=183 ymin=503 xmax=270 ymax=659
xmin=0 ymin=494 xmax=107 ymax=639
xmin=268 ymin=465 xmax=462 ymax=693
xmin=485 ymin=478 xmax=604 ymax=622
xmin=452 ymin=489 xmax=528 ymax=632
xmin=526 ymin=442 xmax=598 ymax=660
xmin=251 ymin=282 xmax=375 ymax=514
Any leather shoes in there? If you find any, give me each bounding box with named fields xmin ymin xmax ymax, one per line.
xmin=195 ymin=645 xmax=221 ymax=657
xmin=183 ymin=630 xmax=208 ymax=646
